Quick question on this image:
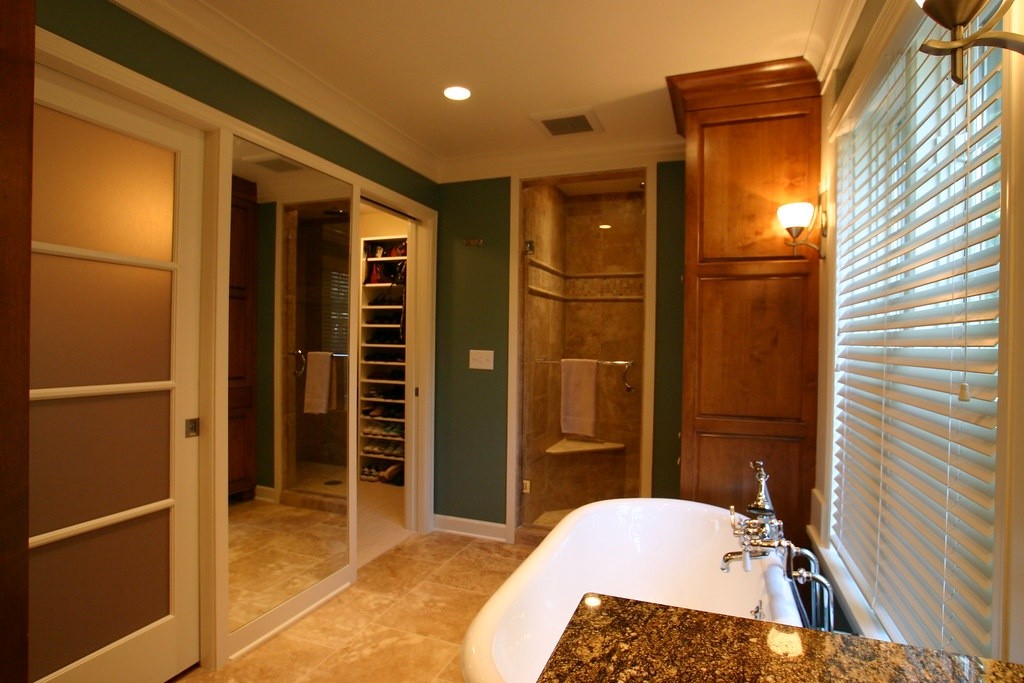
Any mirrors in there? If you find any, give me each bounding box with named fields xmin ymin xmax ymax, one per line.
xmin=228 ymin=133 xmax=354 ymax=637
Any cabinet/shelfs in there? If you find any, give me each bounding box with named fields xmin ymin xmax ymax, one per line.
xmin=666 ymin=54 xmax=823 ymax=623
xmin=355 ymin=234 xmax=408 ymax=485
xmin=227 ymin=172 xmax=257 ymax=501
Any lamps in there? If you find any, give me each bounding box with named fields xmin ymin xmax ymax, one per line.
xmin=915 ymin=0 xmax=1024 ymax=83
xmin=774 ymin=191 xmax=830 ymax=258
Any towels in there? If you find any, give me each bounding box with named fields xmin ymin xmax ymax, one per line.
xmin=301 ymin=350 xmax=338 ymax=417
xmin=560 ymin=356 xmax=599 ymax=439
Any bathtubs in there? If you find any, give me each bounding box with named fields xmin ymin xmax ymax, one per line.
xmin=460 ymin=497 xmax=808 ymax=681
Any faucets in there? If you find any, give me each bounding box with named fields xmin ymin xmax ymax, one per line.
xmin=719 ymin=549 xmax=768 ymax=575
